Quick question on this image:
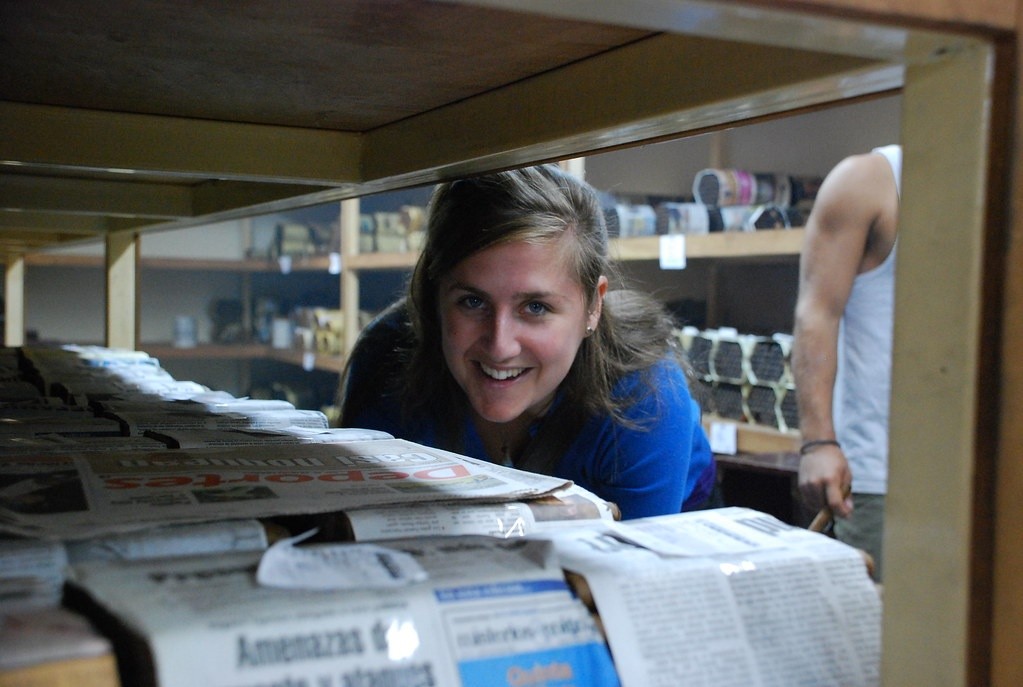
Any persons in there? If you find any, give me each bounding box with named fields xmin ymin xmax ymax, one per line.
xmin=792 ymin=144 xmax=902 ymax=581
xmin=342 ymin=163 xmax=725 ymax=521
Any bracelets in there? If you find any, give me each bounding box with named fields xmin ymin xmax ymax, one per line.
xmin=800 ymin=439 xmax=841 ymax=454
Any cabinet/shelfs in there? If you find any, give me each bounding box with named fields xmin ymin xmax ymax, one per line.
xmin=0 ymin=2 xmax=1022 ymax=687
